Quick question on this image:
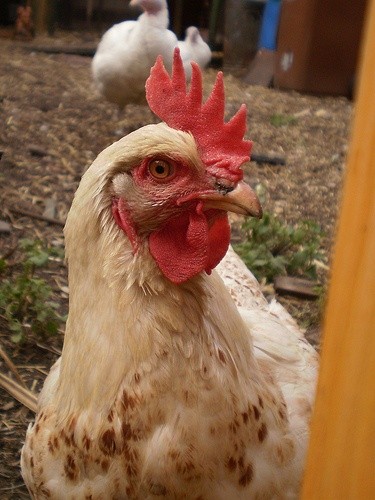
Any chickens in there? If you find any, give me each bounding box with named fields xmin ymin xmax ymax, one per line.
xmin=16 ymin=46 xmax=323 ymax=500
xmin=88 ymin=0 xmax=214 ymax=121
